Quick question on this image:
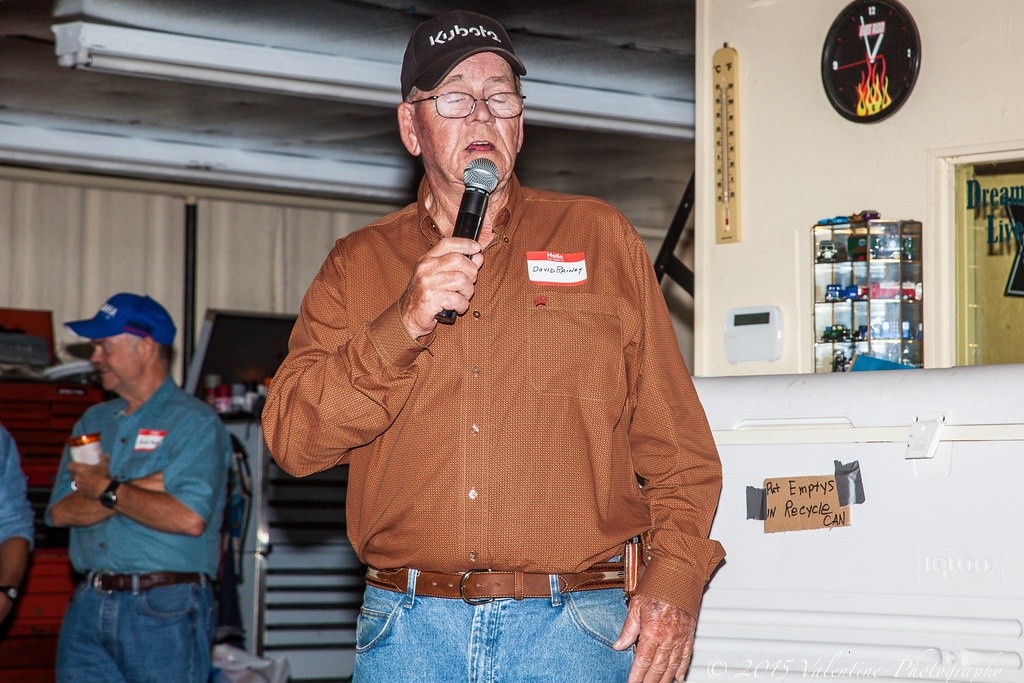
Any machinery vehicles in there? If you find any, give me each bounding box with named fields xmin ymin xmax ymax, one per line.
xmin=858 ymin=281 xmax=916 ymax=300
xmin=847 ymin=232 xmax=912 ymax=260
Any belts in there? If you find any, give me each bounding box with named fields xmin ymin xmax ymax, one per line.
xmin=367 ymin=559 xmax=641 ymax=605
xmin=75 ymin=567 xmax=207 ymax=593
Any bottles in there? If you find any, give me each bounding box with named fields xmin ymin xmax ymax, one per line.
xmin=206 ymin=375 xmax=273 ymax=415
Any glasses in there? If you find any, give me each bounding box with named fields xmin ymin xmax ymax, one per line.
xmin=408 ymin=91 xmax=526 ymax=120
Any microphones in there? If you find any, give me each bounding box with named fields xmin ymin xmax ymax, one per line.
xmin=435 ymin=158 xmax=500 ymax=324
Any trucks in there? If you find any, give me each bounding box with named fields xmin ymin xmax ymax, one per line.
xmin=825 ymin=284 xmax=858 ymax=302
xmin=862 ymin=321 xmax=911 ymax=340
xmin=817 ymin=240 xmax=847 ymax=262
xmin=821 ymin=324 xmax=860 ymax=342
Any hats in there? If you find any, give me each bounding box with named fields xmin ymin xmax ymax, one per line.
xmin=63 ymin=291 xmax=177 ymax=346
xmin=401 ymin=8 xmax=527 ymax=100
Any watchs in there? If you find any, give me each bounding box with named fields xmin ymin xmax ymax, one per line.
xmin=100 ymin=480 xmax=122 ymax=508
xmin=0 ymin=586 xmax=18 ymax=601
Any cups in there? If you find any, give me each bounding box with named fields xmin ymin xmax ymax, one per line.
xmin=68 ymin=434 xmax=104 ymax=466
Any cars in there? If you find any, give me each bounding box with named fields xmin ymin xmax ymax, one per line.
xmin=848 ymin=210 xmax=880 ymax=223
xmin=818 ymin=216 xmax=848 ymax=225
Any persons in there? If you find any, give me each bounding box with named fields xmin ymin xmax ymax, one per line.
xmin=45 ymin=293 xmax=232 ymax=683
xmin=0 ymin=426 xmax=36 ymax=622
xmin=262 ymin=11 xmax=728 ymax=683
